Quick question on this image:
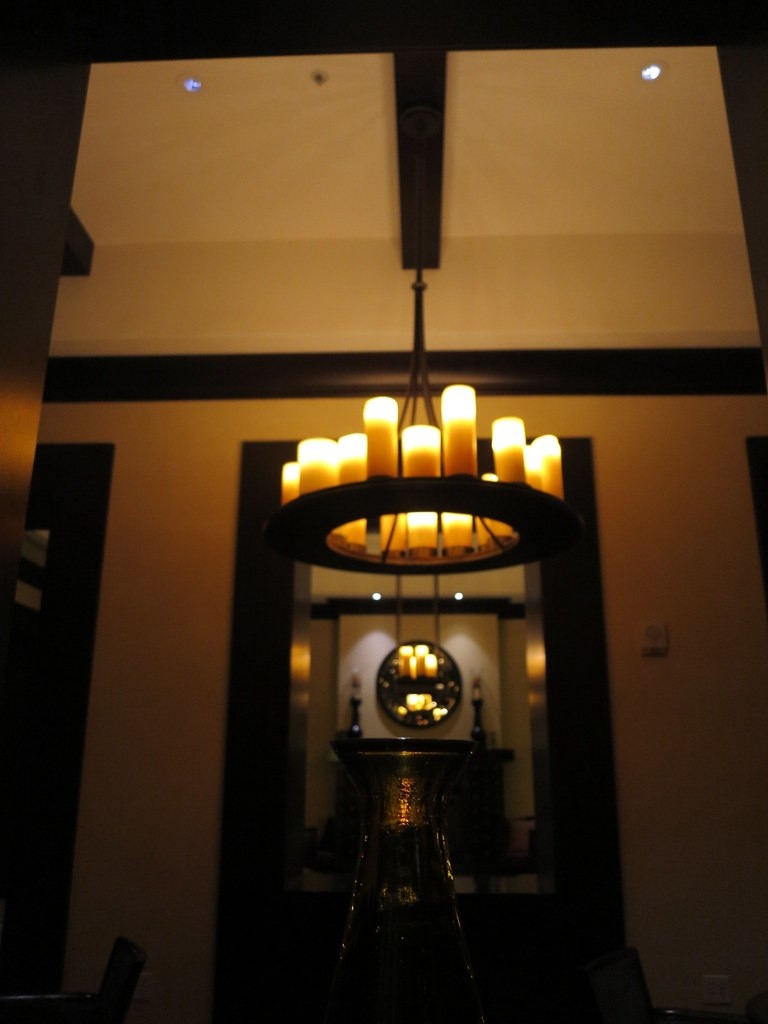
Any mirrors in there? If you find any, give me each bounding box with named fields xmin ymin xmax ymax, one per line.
xmin=216 ymin=440 xmax=624 ymax=1024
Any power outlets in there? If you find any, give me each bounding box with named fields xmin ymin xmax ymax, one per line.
xmin=703 ymin=975 xmax=731 ymax=1004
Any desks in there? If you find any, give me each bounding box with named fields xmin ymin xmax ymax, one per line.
xmin=658 ymin=1007 xmax=750 ymax=1024
xmin=0 ymin=987 xmax=92 ymax=1005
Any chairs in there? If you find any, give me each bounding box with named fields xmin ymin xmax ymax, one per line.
xmin=95 ymin=935 xmax=146 ymax=1024
xmin=585 ymin=947 xmax=654 ymax=1024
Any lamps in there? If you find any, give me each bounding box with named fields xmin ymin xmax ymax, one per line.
xmin=265 ymin=50 xmax=587 ymax=575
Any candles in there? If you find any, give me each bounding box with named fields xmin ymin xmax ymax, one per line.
xmin=352 ymin=679 xmax=361 ymax=696
xmin=472 ymin=677 xmax=479 ymax=700
